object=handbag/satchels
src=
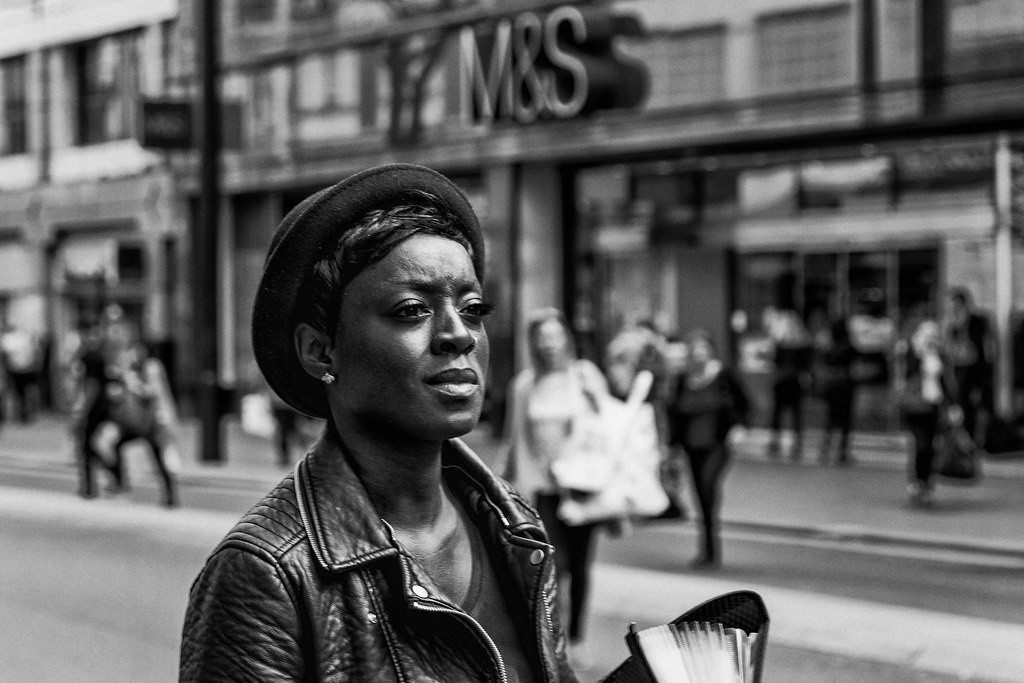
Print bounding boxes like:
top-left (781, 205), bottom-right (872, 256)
top-left (938, 429), bottom-right (980, 480)
top-left (552, 400), bottom-right (668, 528)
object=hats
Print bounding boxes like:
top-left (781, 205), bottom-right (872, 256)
top-left (251, 167), bottom-right (484, 419)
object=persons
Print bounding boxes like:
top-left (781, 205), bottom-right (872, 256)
top-left (178, 163), bottom-right (581, 683)
top-left (270, 392), bottom-right (320, 468)
top-left (632, 285), bottom-right (1022, 575)
top-left (68, 319), bottom-right (181, 509)
top-left (499, 307), bottom-right (613, 673)
